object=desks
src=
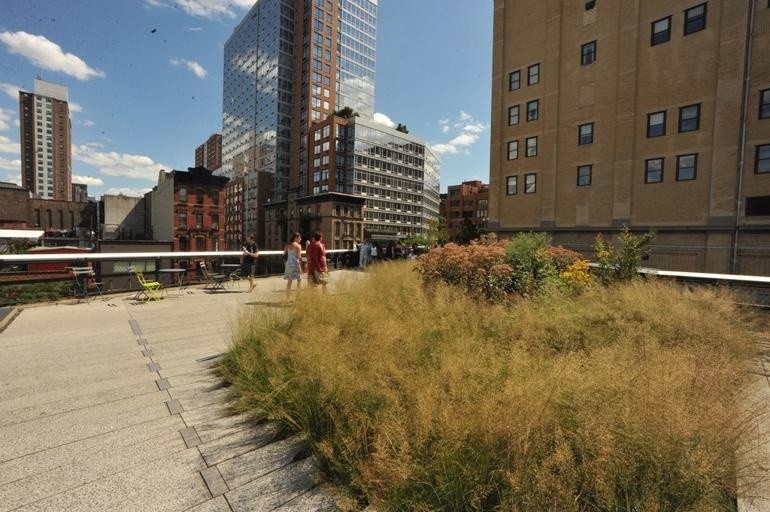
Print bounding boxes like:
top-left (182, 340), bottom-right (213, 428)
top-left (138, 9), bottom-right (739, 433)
top-left (158, 268), bottom-right (188, 299)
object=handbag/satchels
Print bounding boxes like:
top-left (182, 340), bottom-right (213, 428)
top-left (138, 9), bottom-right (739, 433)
top-left (240, 255), bottom-right (246, 264)
top-left (314, 271), bottom-right (329, 285)
top-left (283, 247), bottom-right (288, 261)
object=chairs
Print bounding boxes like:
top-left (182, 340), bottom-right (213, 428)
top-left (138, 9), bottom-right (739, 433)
top-left (124, 266), bottom-right (162, 304)
top-left (197, 260), bottom-right (242, 293)
top-left (64, 265), bottom-right (118, 309)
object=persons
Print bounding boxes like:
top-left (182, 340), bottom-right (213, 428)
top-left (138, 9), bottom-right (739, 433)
top-left (306, 232), bottom-right (329, 289)
top-left (357, 237), bottom-right (422, 269)
top-left (283, 232), bottom-right (303, 304)
top-left (242, 234), bottom-right (259, 294)
